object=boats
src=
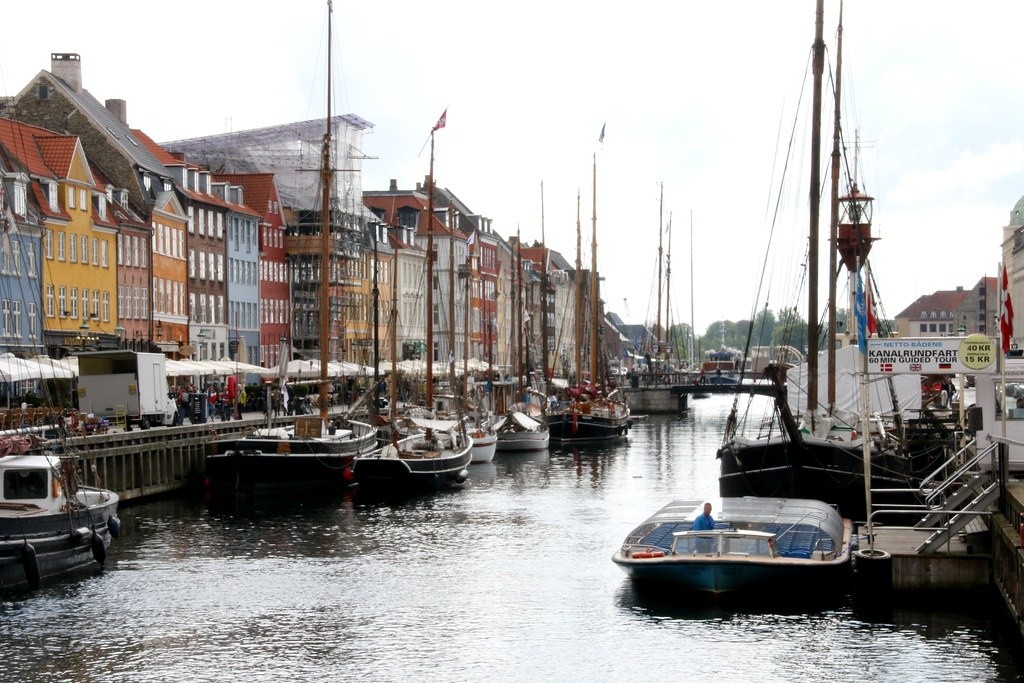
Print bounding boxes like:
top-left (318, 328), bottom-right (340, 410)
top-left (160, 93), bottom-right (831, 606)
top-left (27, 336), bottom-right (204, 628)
top-left (612, 495), bottom-right (855, 616)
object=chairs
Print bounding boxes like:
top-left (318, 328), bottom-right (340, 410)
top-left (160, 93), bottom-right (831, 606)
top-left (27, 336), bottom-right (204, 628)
top-left (0, 406), bottom-right (67, 431)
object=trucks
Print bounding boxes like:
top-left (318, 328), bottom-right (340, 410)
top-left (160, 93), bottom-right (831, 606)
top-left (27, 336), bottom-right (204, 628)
top-left (72, 349), bottom-right (180, 431)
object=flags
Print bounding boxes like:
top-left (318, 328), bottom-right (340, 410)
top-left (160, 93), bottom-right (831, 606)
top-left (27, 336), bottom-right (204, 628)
top-left (999, 265), bottom-right (1015, 354)
top-left (599, 124), bottom-right (605, 143)
top-left (855, 264), bottom-right (879, 353)
top-left (433, 110), bottom-right (445, 131)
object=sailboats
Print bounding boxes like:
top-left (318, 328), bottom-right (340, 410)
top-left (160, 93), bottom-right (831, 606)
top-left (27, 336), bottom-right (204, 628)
top-left (204, 3), bottom-right (379, 519)
top-left (0, 148), bottom-right (122, 593)
top-left (349, 148), bottom-right (711, 466)
top-left (715, 2), bottom-right (924, 526)
top-left (350, 125), bottom-right (474, 505)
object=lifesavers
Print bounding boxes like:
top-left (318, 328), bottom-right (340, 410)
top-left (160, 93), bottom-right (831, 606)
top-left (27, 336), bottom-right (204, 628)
top-left (53, 478), bottom-right (61, 498)
top-left (610, 402), bottom-right (615, 413)
top-left (632, 551), bottom-right (665, 558)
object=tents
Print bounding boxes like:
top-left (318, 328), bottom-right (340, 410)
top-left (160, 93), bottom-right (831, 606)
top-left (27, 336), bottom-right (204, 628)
top-left (0, 357), bottom-right (501, 408)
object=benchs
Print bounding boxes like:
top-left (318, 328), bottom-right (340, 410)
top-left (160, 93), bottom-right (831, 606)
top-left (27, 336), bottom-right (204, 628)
top-left (399, 450), bottom-right (442, 459)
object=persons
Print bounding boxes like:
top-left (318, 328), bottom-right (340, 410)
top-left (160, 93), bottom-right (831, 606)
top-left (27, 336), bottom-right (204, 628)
top-left (692, 503), bottom-right (715, 553)
top-left (942, 377), bottom-right (955, 408)
top-left (714, 368), bottom-right (723, 386)
top-left (171, 378), bottom-right (418, 425)
top-left (699, 368), bottom-right (707, 384)
